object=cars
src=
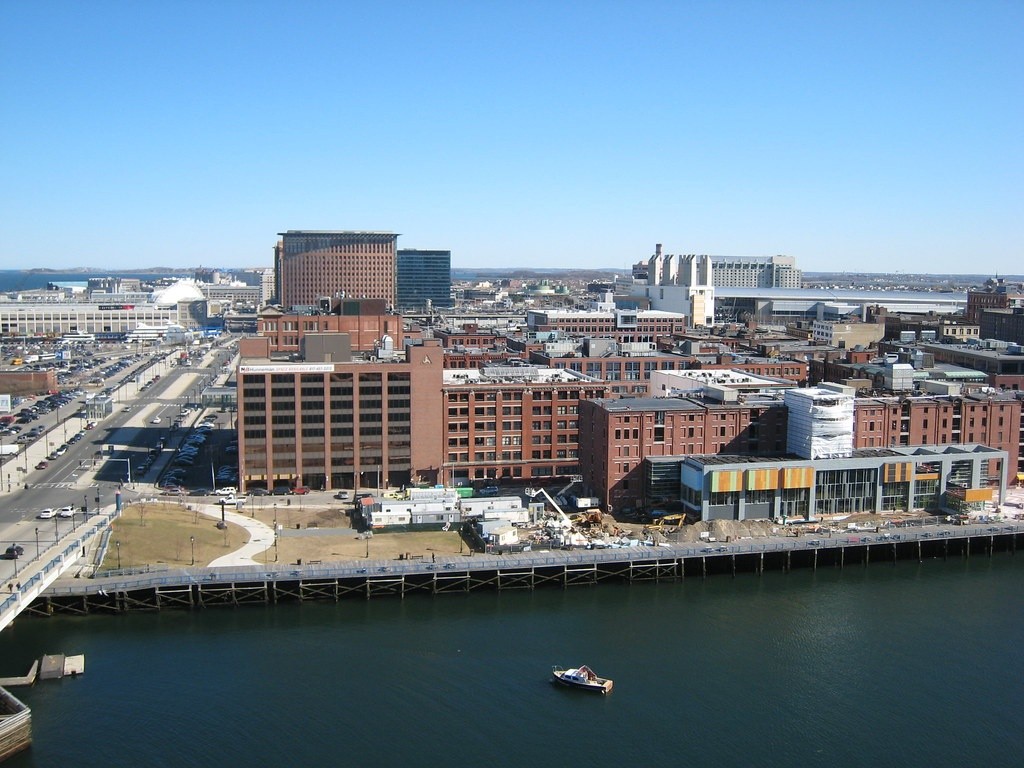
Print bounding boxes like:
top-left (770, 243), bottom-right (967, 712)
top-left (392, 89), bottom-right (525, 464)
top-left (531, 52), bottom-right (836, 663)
top-left (60, 504), bottom-right (76, 520)
top-left (0, 349), bottom-right (239, 496)
top-left (338, 490), bottom-right (349, 499)
top-left (4, 545), bottom-right (24, 560)
top-left (39, 505), bottom-right (55, 518)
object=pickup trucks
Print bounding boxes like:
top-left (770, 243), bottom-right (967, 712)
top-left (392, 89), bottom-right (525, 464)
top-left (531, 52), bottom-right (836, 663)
top-left (219, 494), bottom-right (247, 505)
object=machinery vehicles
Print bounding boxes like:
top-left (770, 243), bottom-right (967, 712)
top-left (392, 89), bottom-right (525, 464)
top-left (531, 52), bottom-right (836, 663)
top-left (523, 486), bottom-right (573, 530)
top-left (643, 513), bottom-right (687, 533)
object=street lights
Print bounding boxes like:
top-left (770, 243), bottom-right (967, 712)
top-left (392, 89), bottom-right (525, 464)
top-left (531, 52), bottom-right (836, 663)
top-left (54, 514), bottom-right (59, 543)
top-left (95, 488), bottom-right (100, 515)
top-left (84, 495), bottom-right (88, 523)
top-left (71, 502), bottom-right (76, 532)
top-left (12, 543), bottom-right (18, 577)
top-left (273, 503), bottom-right (278, 563)
top-left (116, 541), bottom-right (120, 570)
top-left (190, 536), bottom-right (195, 565)
top-left (36, 527), bottom-right (39, 561)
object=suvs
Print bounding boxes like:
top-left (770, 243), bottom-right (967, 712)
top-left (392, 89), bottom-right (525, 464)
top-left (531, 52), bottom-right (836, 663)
top-left (214, 486), bottom-right (236, 496)
top-left (247, 485), bottom-right (310, 498)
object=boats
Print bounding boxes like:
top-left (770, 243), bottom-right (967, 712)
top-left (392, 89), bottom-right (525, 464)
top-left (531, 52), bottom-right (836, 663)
top-left (552, 667), bottom-right (615, 696)
